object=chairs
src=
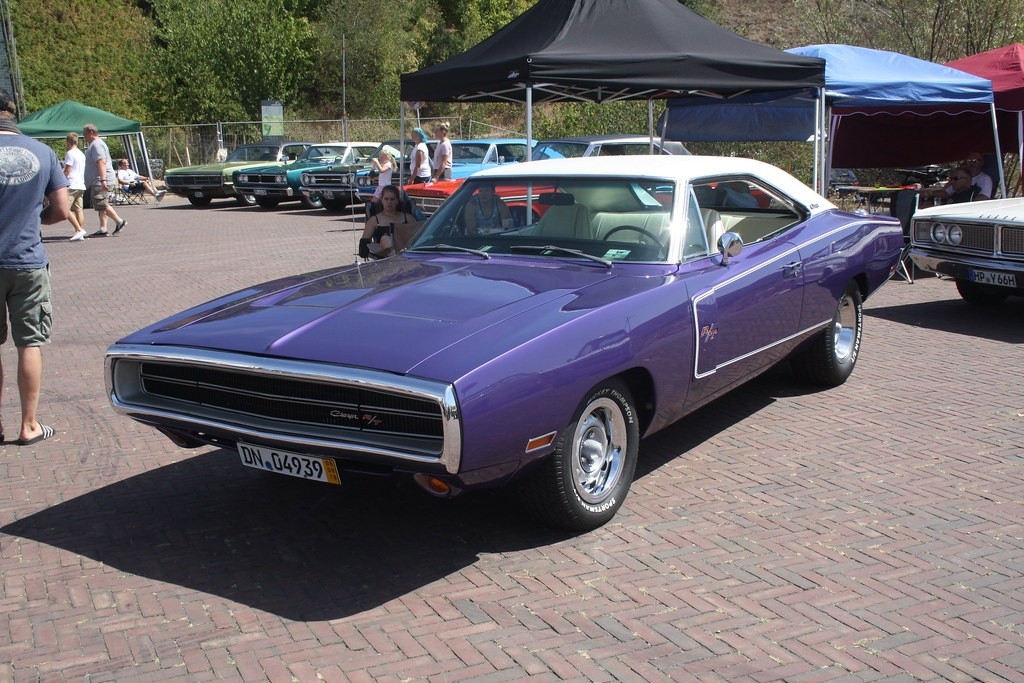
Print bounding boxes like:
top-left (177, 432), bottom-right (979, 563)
top-left (359, 191), bottom-right (426, 263)
top-left (115, 171), bottom-right (148, 205)
top-left (892, 153), bottom-right (1008, 286)
top-left (661, 209), bottom-right (727, 264)
top-left (530, 205), bottom-right (592, 240)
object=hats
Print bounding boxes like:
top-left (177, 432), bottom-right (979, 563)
top-left (0, 88), bottom-right (17, 114)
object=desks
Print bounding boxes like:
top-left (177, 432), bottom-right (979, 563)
top-left (856, 186), bottom-right (909, 213)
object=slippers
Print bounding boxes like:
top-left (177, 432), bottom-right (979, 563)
top-left (17, 421), bottom-right (56, 445)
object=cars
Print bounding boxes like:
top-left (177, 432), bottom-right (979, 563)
top-left (908, 194), bottom-right (1024, 318)
top-left (100, 154), bottom-right (903, 535)
top-left (353, 138), bottom-right (566, 204)
top-left (829, 168), bottom-right (861, 197)
top-left (163, 142), bottom-right (337, 207)
top-left (231, 142), bottom-right (408, 209)
top-left (397, 135), bottom-right (771, 231)
top-left (298, 139), bottom-right (415, 214)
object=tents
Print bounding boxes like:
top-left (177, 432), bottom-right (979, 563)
top-left (944, 41), bottom-right (1024, 177)
top-left (12, 98), bottom-right (160, 204)
top-left (398, 0), bottom-right (827, 225)
top-left (656, 43), bottom-right (1007, 198)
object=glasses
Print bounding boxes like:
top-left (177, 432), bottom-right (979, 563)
top-left (966, 159), bottom-right (976, 165)
top-left (949, 177), bottom-right (967, 182)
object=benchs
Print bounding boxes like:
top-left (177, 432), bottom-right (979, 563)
top-left (593, 210), bottom-right (789, 261)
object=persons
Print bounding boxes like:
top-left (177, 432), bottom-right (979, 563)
top-left (465, 183), bottom-right (512, 235)
top-left (370, 150), bottom-right (398, 202)
top-left (932, 147), bottom-right (993, 206)
top-left (62, 124), bottom-right (128, 241)
top-left (117, 159), bottom-right (166, 202)
top-left (0, 87), bottom-right (70, 445)
top-left (359, 185), bottom-right (418, 259)
top-left (432, 121), bottom-right (452, 180)
top-left (407, 127), bottom-right (431, 184)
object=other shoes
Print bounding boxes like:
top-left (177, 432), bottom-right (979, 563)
top-left (112, 220), bottom-right (126, 236)
top-left (70, 234), bottom-right (84, 241)
top-left (90, 230), bottom-right (109, 238)
top-left (157, 190), bottom-right (167, 202)
